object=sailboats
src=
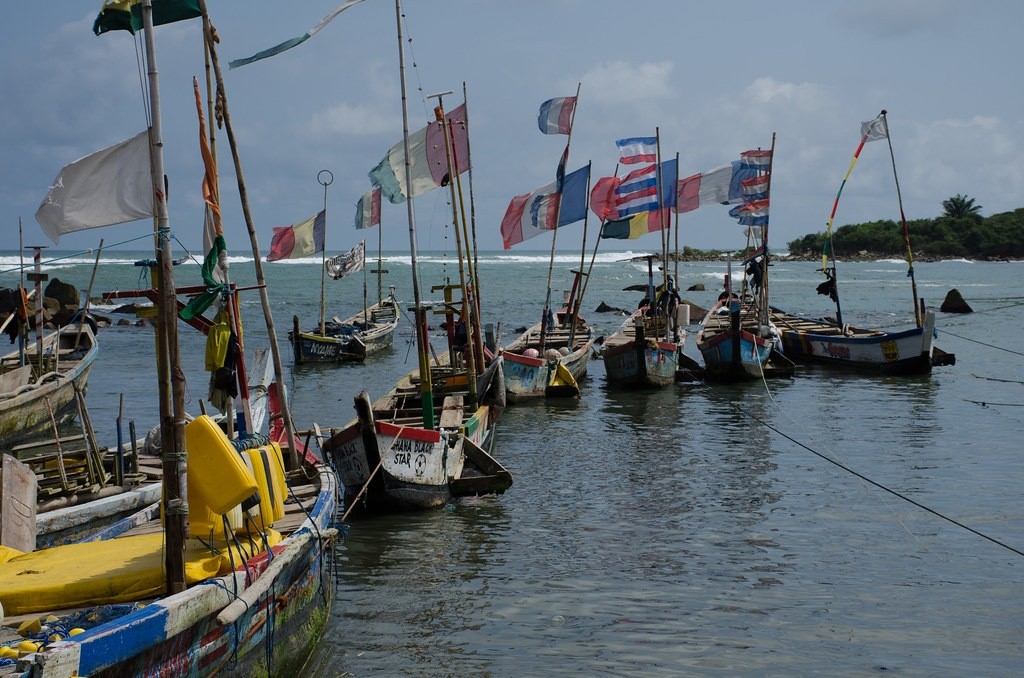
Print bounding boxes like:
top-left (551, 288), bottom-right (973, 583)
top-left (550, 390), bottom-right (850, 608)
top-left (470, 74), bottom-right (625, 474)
top-left (502, 83), bottom-right (619, 405)
top-left (322, 0), bottom-right (513, 514)
top-left (598, 127), bottom-right (686, 392)
top-left (695, 132), bottom-right (784, 382)
top-left (0, 0), bottom-right (338, 678)
top-left (767, 109), bottom-right (937, 378)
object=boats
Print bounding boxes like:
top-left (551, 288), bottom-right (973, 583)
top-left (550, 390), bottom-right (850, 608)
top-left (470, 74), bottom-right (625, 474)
top-left (267, 170), bottom-right (399, 366)
top-left (87, 302), bottom-right (154, 328)
top-left (0, 216), bottom-right (99, 449)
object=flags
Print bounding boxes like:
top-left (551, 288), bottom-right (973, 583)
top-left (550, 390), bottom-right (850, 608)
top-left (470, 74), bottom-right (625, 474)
top-left (368, 103), bottom-right (470, 204)
top-left (743, 226), bottom-right (762, 239)
top-left (34, 127), bottom-right (154, 245)
top-left (737, 199), bottom-right (769, 225)
top-left (861, 115), bottom-right (888, 143)
top-left (176, 88), bottom-right (240, 416)
top-left (538, 95), bottom-right (577, 135)
top-left (500, 163), bottom-right (590, 251)
top-left (556, 145), bottom-right (568, 193)
top-left (92, 0), bottom-right (204, 37)
top-left (740, 174), bottom-right (769, 202)
top-left (728, 203), bottom-right (747, 218)
top-left (326, 238), bottom-right (365, 280)
top-left (615, 137), bottom-right (657, 164)
top-left (741, 244), bottom-right (764, 267)
top-left (740, 149), bottom-right (771, 171)
top-left (671, 159), bottom-right (757, 214)
top-left (591, 157), bottom-right (676, 223)
top-left (600, 198), bottom-right (670, 240)
top-left (354, 186), bottom-right (379, 230)
top-left (266, 211), bottom-right (324, 262)
